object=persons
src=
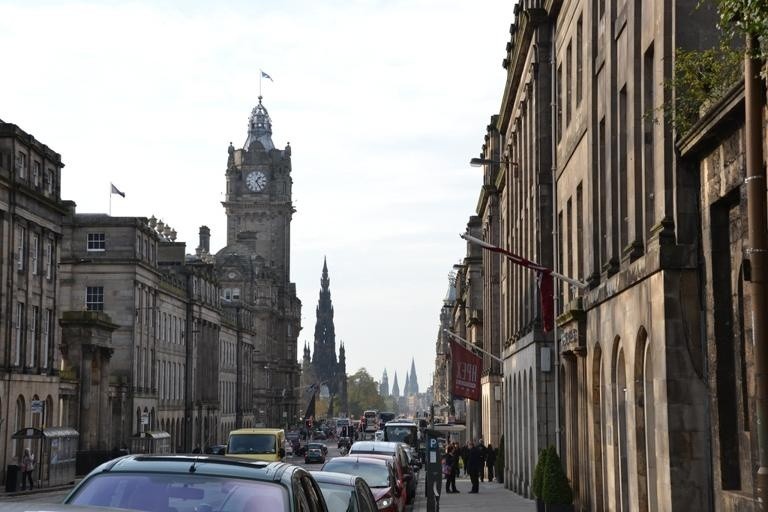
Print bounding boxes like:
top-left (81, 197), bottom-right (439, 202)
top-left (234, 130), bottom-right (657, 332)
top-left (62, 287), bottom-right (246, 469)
top-left (443, 438), bottom-right (496, 494)
top-left (21, 446), bottom-right (35, 491)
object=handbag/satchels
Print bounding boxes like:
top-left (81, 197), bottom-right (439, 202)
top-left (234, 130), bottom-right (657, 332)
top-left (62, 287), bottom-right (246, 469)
top-left (442, 464), bottom-right (452, 475)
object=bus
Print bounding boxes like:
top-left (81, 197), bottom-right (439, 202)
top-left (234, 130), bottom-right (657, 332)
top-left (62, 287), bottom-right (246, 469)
top-left (224, 428), bottom-right (286, 462)
top-left (380, 412), bottom-right (428, 451)
top-left (363, 411), bottom-right (378, 432)
top-left (336, 419), bottom-right (351, 437)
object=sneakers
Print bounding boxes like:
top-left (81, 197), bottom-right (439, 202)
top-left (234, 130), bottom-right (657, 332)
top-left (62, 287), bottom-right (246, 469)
top-left (468, 489), bottom-right (479, 494)
top-left (446, 488), bottom-right (460, 494)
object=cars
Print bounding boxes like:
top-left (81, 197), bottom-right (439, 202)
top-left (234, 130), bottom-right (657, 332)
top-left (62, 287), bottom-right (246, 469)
top-left (310, 442), bottom-right (422, 512)
top-left (287, 433), bottom-right (328, 463)
top-left (63, 452), bottom-right (328, 512)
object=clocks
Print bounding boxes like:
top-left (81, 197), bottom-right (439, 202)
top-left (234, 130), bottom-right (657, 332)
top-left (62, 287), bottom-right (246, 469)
top-left (245, 169), bottom-right (268, 193)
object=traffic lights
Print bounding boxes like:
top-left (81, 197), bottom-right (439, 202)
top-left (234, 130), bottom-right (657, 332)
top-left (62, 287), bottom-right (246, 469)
top-left (305, 420), bottom-right (312, 428)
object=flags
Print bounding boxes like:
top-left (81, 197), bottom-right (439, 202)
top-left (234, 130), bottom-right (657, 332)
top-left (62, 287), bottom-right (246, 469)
top-left (111, 183), bottom-right (126, 199)
top-left (449, 338), bottom-right (482, 402)
top-left (262, 71), bottom-right (273, 83)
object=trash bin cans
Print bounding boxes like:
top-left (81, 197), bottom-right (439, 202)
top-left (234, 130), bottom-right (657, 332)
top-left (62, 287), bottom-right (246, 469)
top-left (6, 465), bottom-right (23, 492)
